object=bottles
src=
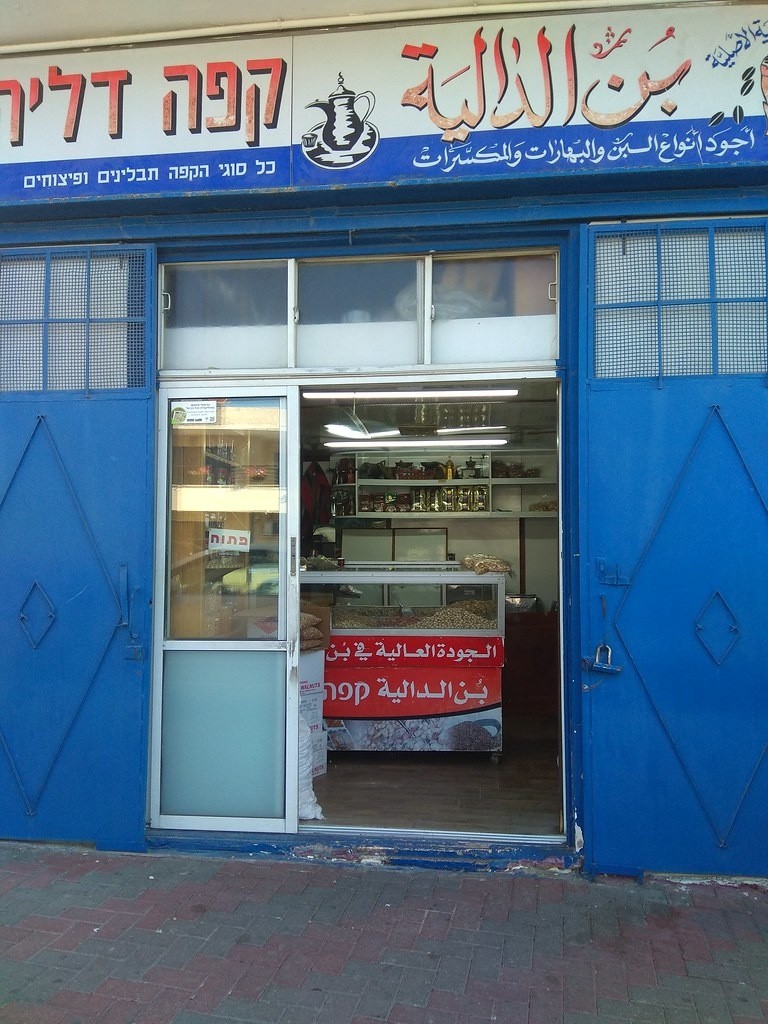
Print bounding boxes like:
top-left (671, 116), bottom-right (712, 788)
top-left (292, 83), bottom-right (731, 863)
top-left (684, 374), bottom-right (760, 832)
top-left (445, 456), bottom-right (453, 480)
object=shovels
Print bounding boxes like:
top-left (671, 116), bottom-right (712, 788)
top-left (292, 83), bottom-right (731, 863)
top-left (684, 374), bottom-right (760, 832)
top-left (392, 591), bottom-right (414, 617)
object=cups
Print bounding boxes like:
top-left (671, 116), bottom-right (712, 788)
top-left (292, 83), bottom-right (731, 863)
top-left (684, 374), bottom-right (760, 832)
top-left (338, 558), bottom-right (345, 567)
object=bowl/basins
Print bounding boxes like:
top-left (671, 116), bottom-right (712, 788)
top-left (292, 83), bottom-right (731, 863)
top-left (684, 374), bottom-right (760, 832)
top-left (465, 461), bottom-right (476, 467)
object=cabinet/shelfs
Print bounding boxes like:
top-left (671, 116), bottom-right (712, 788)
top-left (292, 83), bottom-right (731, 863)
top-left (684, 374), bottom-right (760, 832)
top-left (327, 449), bottom-right (559, 520)
top-left (167, 429), bottom-right (252, 642)
